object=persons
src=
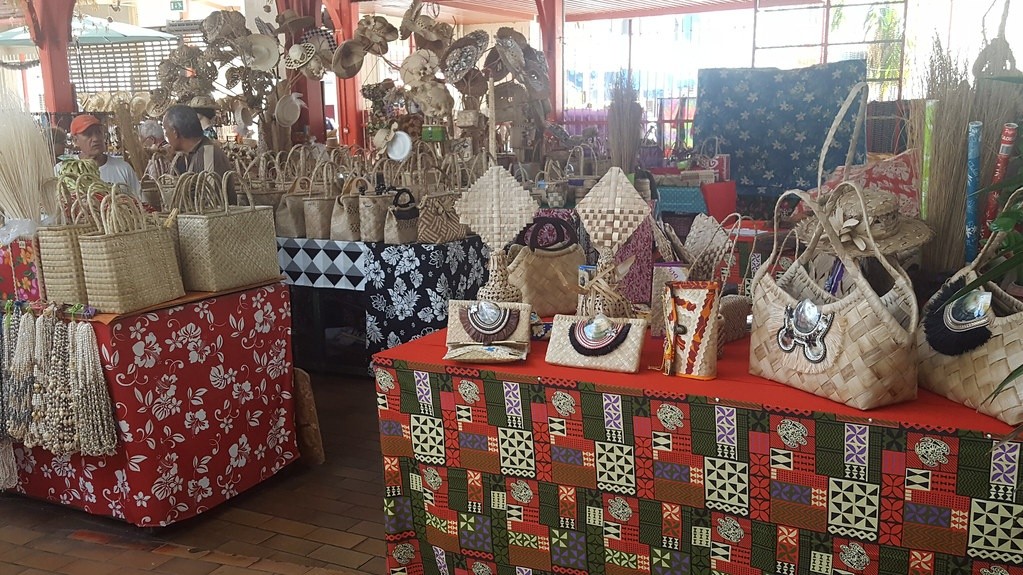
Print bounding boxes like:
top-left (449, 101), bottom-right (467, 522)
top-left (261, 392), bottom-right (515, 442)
top-left (42, 126), bottom-right (67, 165)
top-left (139, 120), bottom-right (171, 149)
top-left (54, 115), bottom-right (144, 204)
top-left (163, 104), bottom-right (238, 206)
top-left (188, 95), bottom-right (218, 142)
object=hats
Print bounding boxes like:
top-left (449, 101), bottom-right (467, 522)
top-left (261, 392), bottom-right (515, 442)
top-left (85, 8), bottom-right (556, 161)
top-left (70, 115), bottom-right (101, 135)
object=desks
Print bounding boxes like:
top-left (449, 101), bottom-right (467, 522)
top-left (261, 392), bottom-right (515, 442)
top-left (0, 181), bottom-right (1023, 575)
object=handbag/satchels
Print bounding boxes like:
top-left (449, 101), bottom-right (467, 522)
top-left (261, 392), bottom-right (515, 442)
top-left (253, 82), bottom-right (1023, 427)
top-left (38, 172), bottom-right (279, 316)
